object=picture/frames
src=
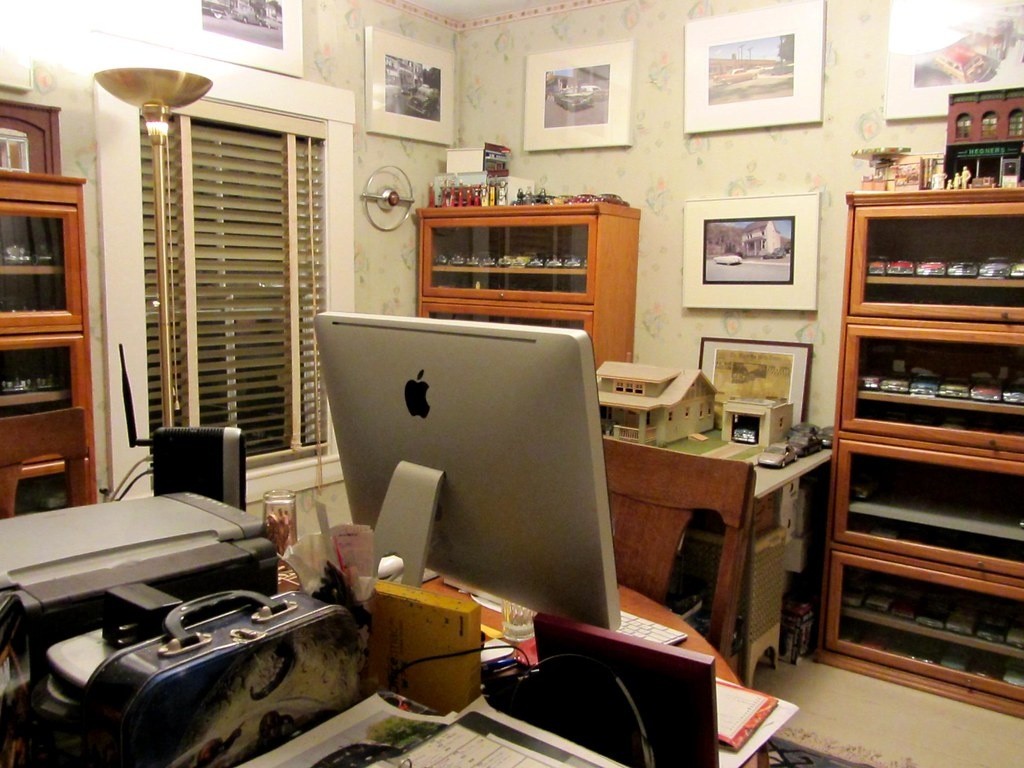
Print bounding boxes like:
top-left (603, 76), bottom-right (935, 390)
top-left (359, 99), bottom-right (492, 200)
top-left (684, 2), bottom-right (828, 134)
top-left (523, 37), bottom-right (635, 151)
top-left (698, 337), bottom-right (813, 428)
top-left (200, 0), bottom-right (302, 78)
top-left (683, 193), bottom-right (818, 310)
top-left (364, 25), bottom-right (452, 145)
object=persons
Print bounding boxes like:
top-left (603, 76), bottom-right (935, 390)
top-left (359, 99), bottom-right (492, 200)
top-left (946, 166), bottom-right (971, 189)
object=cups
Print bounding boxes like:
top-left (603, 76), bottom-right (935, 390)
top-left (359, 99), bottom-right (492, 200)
top-left (261, 489), bottom-right (298, 570)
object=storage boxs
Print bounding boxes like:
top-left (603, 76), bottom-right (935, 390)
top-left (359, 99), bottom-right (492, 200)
top-left (777, 597), bottom-right (817, 664)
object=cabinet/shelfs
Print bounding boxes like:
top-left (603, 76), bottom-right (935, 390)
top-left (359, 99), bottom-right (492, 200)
top-left (414, 201), bottom-right (641, 374)
top-left (816, 187), bottom-right (1024, 716)
top-left (0, 168), bottom-right (98, 509)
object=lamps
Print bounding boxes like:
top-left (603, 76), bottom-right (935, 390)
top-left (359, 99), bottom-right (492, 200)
top-left (97, 67), bottom-right (215, 425)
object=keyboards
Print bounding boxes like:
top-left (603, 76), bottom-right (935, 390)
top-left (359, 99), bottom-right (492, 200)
top-left (442, 577), bottom-right (688, 648)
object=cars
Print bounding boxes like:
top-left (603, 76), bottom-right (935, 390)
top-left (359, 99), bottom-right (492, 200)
top-left (859, 372), bottom-right (1024, 404)
top-left (841, 594), bottom-right (1024, 651)
top-left (202, 0), bottom-right (282, 31)
top-left (510, 193), bottom-right (630, 206)
top-left (757, 246), bottom-right (786, 260)
top-left (869, 255), bottom-right (1024, 278)
top-left (405, 83), bottom-right (440, 119)
top-left (714, 66), bottom-right (767, 84)
top-left (713, 253), bottom-right (742, 265)
top-left (554, 85), bottom-right (601, 113)
top-left (434, 254), bottom-right (587, 269)
top-left (757, 424), bottom-right (834, 469)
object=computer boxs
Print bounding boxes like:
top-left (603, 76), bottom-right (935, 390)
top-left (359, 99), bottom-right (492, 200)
top-left (151, 426), bottom-right (246, 512)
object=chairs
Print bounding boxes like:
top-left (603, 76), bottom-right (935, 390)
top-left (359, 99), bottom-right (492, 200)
top-left (2, 408), bottom-right (90, 518)
top-left (110, 342), bottom-right (154, 500)
top-left (603, 435), bottom-right (756, 673)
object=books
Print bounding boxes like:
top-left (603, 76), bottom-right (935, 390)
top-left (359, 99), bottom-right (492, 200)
top-left (716, 681), bottom-right (778, 752)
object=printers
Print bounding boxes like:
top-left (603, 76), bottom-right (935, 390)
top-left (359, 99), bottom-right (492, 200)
top-left (0, 492), bottom-right (280, 738)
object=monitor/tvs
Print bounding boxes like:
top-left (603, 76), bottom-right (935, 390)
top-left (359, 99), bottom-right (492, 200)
top-left (314, 310), bottom-right (622, 635)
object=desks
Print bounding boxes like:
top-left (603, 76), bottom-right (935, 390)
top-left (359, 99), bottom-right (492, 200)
top-left (276, 540), bottom-right (770, 768)
top-left (655, 427), bottom-right (834, 675)
